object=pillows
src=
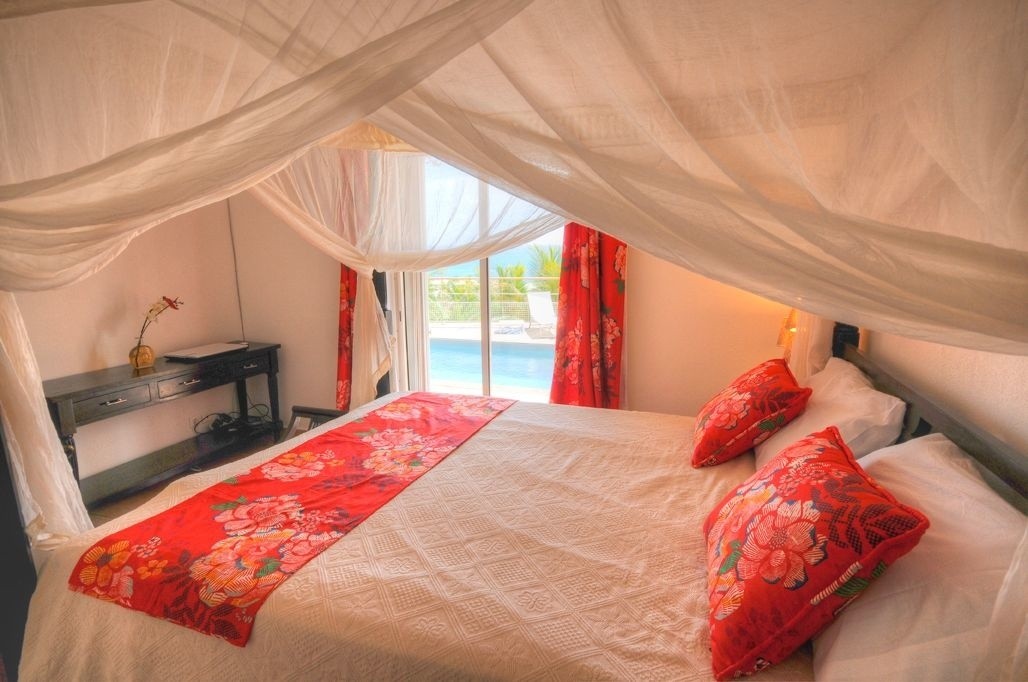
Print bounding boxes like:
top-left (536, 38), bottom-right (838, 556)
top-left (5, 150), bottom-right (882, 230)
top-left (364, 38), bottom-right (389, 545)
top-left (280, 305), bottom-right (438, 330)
top-left (701, 426), bottom-right (929, 681)
top-left (752, 357), bottom-right (908, 478)
top-left (692, 357), bottom-right (813, 469)
top-left (809, 433), bottom-right (1027, 682)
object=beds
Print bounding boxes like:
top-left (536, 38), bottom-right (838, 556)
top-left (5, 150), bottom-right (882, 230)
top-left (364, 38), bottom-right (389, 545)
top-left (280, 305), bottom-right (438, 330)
top-left (0, 319), bottom-right (1027, 682)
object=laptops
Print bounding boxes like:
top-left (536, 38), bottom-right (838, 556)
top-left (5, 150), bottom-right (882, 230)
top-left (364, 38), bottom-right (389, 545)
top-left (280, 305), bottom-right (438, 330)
top-left (164, 343), bottom-right (248, 361)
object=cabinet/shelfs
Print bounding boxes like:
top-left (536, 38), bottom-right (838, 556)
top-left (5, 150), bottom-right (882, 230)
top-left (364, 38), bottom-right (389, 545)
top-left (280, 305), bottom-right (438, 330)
top-left (39, 340), bottom-right (282, 516)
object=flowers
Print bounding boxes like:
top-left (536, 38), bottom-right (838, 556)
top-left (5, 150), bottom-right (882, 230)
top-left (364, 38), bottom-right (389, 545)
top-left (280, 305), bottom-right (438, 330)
top-left (133, 293), bottom-right (184, 366)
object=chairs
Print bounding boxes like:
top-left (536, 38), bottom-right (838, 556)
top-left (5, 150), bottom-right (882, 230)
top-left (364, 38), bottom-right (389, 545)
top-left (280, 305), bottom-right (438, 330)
top-left (527, 289), bottom-right (557, 339)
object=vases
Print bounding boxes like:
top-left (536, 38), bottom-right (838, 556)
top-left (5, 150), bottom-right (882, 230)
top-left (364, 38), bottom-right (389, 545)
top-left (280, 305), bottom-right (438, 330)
top-left (129, 336), bottom-right (156, 368)
